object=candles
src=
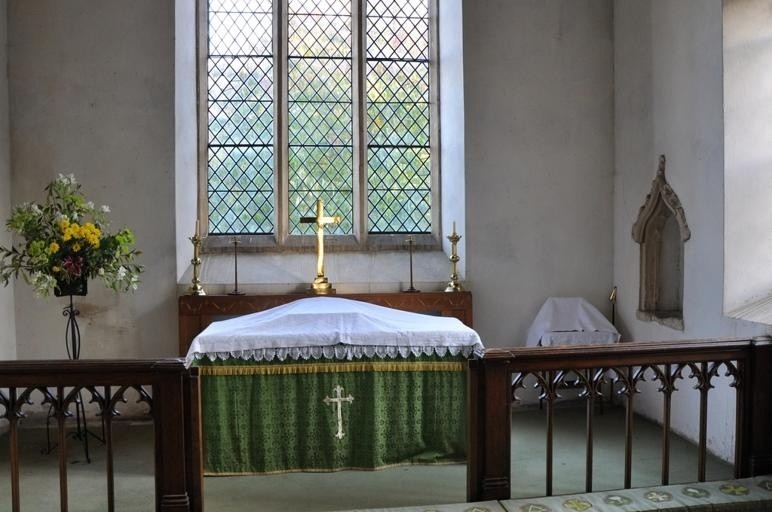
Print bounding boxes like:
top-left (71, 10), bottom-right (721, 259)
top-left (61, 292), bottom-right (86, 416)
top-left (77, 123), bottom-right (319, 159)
top-left (195, 219), bottom-right (200, 237)
top-left (451, 220), bottom-right (457, 235)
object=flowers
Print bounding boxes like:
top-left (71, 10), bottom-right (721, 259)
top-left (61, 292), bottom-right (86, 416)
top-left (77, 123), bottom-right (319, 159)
top-left (0, 170), bottom-right (147, 301)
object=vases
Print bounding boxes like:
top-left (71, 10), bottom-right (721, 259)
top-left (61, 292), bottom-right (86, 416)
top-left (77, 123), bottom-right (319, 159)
top-left (44, 256), bottom-right (95, 298)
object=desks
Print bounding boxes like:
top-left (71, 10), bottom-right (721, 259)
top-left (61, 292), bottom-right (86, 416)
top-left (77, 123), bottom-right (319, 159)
top-left (533, 328), bottom-right (619, 416)
